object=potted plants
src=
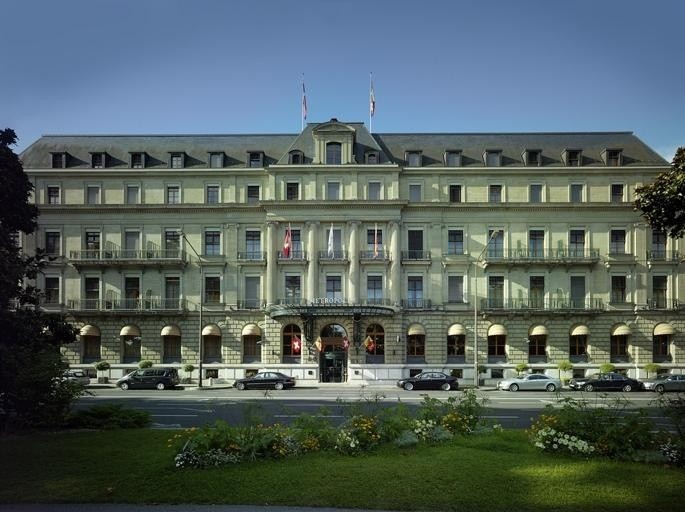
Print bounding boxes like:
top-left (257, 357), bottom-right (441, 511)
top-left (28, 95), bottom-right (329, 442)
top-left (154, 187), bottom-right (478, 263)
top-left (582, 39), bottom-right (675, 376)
top-left (559, 360), bottom-right (573, 386)
top-left (477, 365), bottom-right (487, 387)
top-left (94, 361), bottom-right (110, 384)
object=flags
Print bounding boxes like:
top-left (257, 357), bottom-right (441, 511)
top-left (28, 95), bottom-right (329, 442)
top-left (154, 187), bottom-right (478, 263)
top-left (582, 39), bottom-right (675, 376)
top-left (290, 336), bottom-right (302, 352)
top-left (314, 336), bottom-right (326, 352)
top-left (342, 336), bottom-right (350, 350)
top-left (368, 74), bottom-right (376, 120)
top-left (373, 223), bottom-right (379, 257)
top-left (362, 335), bottom-right (376, 352)
top-left (283, 223), bottom-right (292, 257)
top-left (326, 223), bottom-right (334, 256)
top-left (301, 82), bottom-right (308, 121)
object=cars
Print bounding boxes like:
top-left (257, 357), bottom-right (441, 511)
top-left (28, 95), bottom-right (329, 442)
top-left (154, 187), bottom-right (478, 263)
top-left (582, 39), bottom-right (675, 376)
top-left (232, 370), bottom-right (294, 390)
top-left (57, 368), bottom-right (91, 386)
top-left (641, 374), bottom-right (685, 394)
top-left (569, 370), bottom-right (640, 393)
top-left (498, 373), bottom-right (561, 392)
top-left (396, 372), bottom-right (459, 391)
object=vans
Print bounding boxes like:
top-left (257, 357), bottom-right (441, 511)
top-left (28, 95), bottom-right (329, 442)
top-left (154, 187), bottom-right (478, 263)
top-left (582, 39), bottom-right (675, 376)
top-left (115, 368), bottom-right (180, 391)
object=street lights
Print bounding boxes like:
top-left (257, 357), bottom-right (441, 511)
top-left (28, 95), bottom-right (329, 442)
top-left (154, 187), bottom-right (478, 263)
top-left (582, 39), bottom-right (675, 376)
top-left (175, 227), bottom-right (206, 388)
top-left (470, 226), bottom-right (500, 390)
